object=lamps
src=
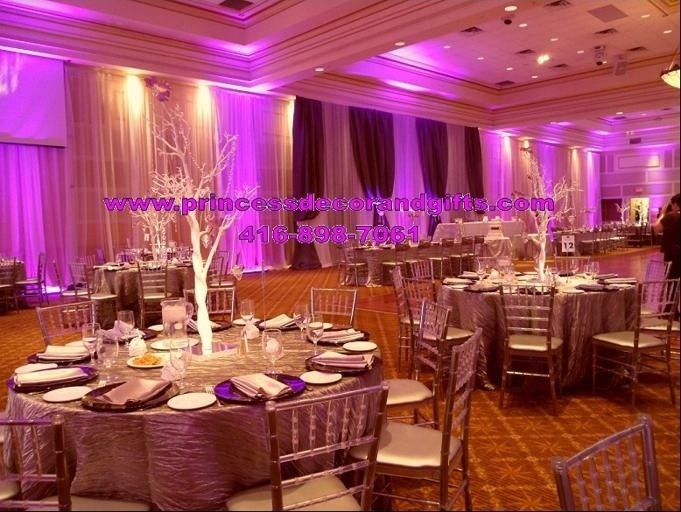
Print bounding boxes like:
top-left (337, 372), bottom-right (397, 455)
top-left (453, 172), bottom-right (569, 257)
top-left (659, 41), bottom-right (679, 88)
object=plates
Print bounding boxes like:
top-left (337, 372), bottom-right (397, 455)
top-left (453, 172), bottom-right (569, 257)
top-left (215, 373), bottom-right (305, 404)
top-left (27, 346), bottom-right (92, 365)
top-left (186, 319), bottom-right (234, 333)
top-left (301, 370), bottom-right (343, 385)
top-left (14, 362), bottom-right (57, 373)
top-left (305, 327), bottom-right (370, 344)
top-left (166, 391), bottom-right (217, 409)
top-left (233, 318), bottom-right (260, 325)
top-left (126, 353), bottom-right (183, 368)
top-left (459, 273), bottom-right (480, 280)
top-left (42, 384), bottom-right (93, 404)
top-left (147, 322), bottom-right (183, 332)
top-left (342, 340), bottom-right (379, 352)
top-left (150, 337), bottom-right (199, 350)
top-left (304, 321), bottom-right (334, 330)
top-left (6, 365), bottom-right (97, 395)
top-left (305, 352), bottom-right (383, 374)
top-left (82, 378), bottom-right (179, 413)
top-left (254, 317), bottom-right (299, 331)
top-left (466, 282), bottom-right (501, 292)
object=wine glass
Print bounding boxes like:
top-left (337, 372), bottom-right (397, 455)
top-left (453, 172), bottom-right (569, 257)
top-left (117, 310), bottom-right (135, 368)
top-left (307, 319), bottom-right (324, 355)
top-left (293, 304), bottom-right (311, 343)
top-left (261, 329), bottom-right (286, 371)
top-left (101, 248), bottom-right (195, 268)
top-left (169, 336), bottom-right (192, 395)
top-left (580, 220), bottom-right (634, 232)
top-left (240, 299), bottom-right (255, 327)
top-left (476, 251), bottom-right (638, 294)
top-left (82, 323), bottom-right (101, 364)
top-left (96, 334), bottom-right (118, 375)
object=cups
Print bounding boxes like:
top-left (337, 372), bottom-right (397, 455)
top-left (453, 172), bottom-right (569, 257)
top-left (161, 297), bottom-right (194, 338)
top-left (483, 214), bottom-right (502, 222)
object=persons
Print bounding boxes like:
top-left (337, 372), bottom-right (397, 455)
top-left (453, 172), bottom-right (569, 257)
top-left (654, 193), bottom-right (680, 321)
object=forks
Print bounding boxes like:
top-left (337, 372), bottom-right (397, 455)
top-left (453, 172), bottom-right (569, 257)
top-left (202, 382), bottom-right (225, 408)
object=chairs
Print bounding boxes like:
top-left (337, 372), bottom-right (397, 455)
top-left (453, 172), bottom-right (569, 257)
top-left (1, 224), bottom-right (681, 511)
